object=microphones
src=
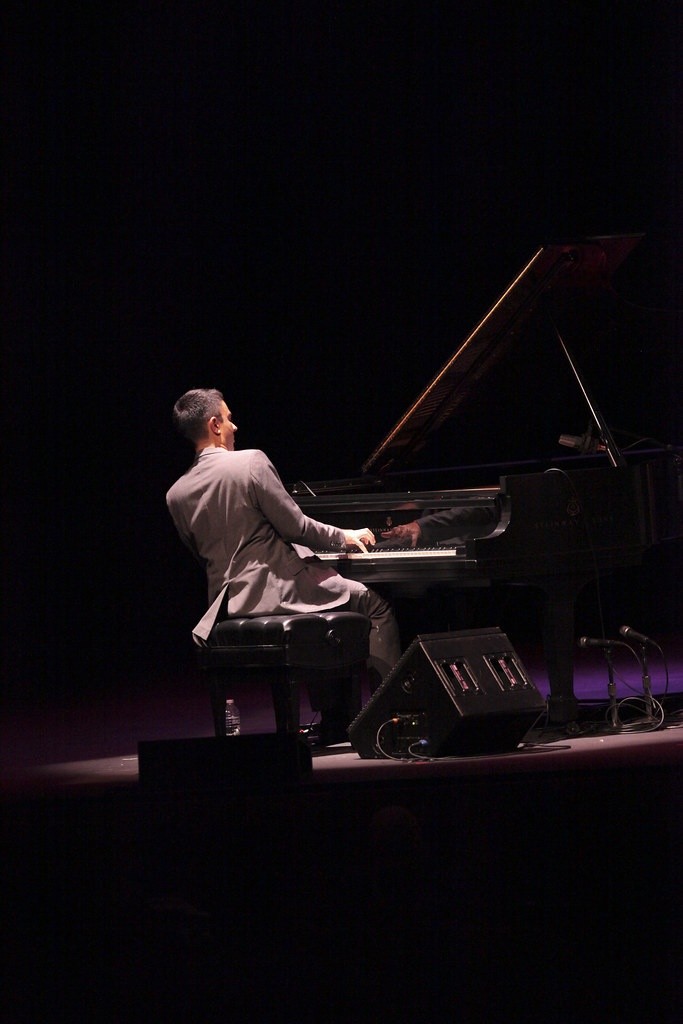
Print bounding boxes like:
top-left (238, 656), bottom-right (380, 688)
top-left (559, 433), bottom-right (609, 456)
top-left (619, 626), bottom-right (658, 647)
top-left (580, 636), bottom-right (626, 649)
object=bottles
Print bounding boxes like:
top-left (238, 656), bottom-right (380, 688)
top-left (226, 699), bottom-right (240, 736)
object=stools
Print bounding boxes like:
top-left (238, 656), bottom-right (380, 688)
top-left (197, 609), bottom-right (372, 753)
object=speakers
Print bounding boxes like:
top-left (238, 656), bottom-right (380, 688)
top-left (346, 627), bottom-right (549, 760)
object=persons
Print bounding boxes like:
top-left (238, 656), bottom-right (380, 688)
top-left (382, 505), bottom-right (491, 548)
top-left (165, 389), bottom-right (400, 711)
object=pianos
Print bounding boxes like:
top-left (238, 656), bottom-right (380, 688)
top-left (282, 232), bottom-right (683, 737)
